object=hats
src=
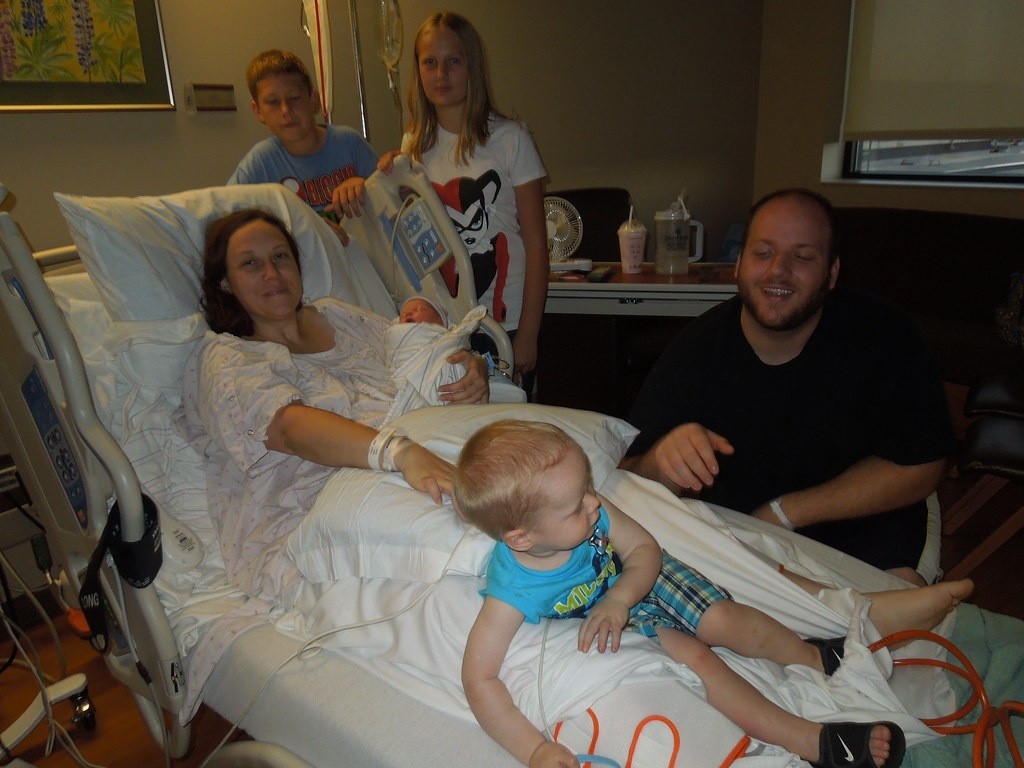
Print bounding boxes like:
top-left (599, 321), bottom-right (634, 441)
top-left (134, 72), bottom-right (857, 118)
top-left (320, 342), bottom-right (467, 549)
top-left (400, 295), bottom-right (449, 330)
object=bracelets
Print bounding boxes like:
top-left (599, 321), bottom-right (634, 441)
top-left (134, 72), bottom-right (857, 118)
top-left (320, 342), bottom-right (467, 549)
top-left (368, 427), bottom-right (411, 471)
top-left (770, 495), bottom-right (795, 531)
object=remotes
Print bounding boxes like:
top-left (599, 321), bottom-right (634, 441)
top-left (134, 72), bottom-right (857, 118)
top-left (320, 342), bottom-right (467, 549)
top-left (550, 258), bottom-right (592, 272)
top-left (586, 266), bottom-right (611, 283)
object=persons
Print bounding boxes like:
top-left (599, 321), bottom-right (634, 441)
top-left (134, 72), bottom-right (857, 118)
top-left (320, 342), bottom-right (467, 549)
top-left (385, 293), bottom-right (490, 406)
top-left (379, 12), bottom-right (549, 389)
top-left (227, 50), bottom-right (378, 247)
top-left (624, 190), bottom-right (948, 590)
top-left (184, 212), bottom-right (973, 653)
top-left (454, 420), bottom-right (908, 768)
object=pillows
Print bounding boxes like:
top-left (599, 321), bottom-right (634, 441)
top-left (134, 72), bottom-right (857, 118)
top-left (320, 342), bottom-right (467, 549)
top-left (54, 182), bottom-right (366, 409)
top-left (259, 400), bottom-right (641, 586)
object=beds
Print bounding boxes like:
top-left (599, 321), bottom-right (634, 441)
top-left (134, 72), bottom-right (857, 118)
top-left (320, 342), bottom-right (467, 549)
top-left (0, 155), bottom-right (1024, 768)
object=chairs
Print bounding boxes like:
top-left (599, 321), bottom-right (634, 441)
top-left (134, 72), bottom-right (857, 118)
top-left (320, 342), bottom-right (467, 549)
top-left (542, 187), bottom-right (639, 270)
top-left (953, 346), bottom-right (1024, 482)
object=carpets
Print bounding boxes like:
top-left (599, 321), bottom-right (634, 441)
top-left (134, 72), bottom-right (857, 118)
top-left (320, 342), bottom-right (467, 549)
top-left (920, 379), bottom-right (1024, 591)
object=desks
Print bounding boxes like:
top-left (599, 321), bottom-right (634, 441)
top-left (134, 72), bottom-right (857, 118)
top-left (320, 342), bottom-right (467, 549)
top-left (528, 262), bottom-right (744, 397)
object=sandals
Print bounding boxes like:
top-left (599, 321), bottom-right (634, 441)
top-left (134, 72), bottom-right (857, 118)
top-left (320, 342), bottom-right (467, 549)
top-left (800, 721), bottom-right (906, 768)
top-left (803, 636), bottom-right (846, 676)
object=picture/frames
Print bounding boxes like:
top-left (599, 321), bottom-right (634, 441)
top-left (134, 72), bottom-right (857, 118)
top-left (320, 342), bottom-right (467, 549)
top-left (0, 0), bottom-right (177, 114)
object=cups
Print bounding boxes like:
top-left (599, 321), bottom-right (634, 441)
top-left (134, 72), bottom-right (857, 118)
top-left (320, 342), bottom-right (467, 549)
top-left (653, 210), bottom-right (704, 276)
top-left (618, 220), bottom-right (647, 274)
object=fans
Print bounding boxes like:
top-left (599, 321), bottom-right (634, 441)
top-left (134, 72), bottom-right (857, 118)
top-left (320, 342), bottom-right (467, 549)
top-left (542, 195), bottom-right (584, 263)
